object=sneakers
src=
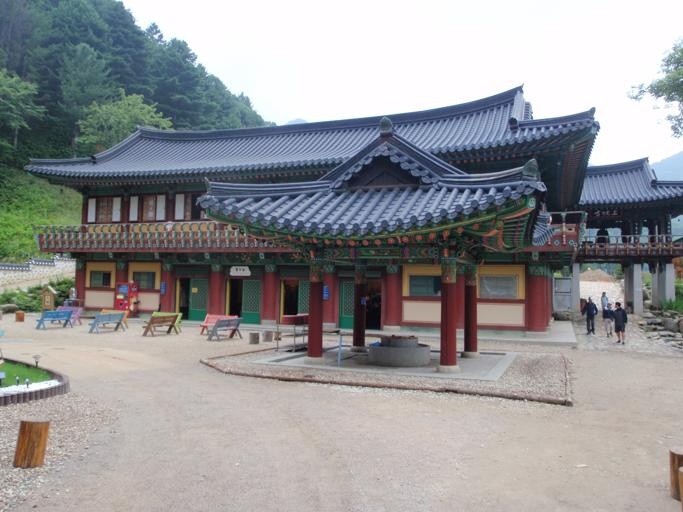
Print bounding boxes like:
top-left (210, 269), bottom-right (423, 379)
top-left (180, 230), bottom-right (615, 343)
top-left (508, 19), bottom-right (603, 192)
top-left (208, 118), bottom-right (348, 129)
top-left (586, 329), bottom-right (625, 345)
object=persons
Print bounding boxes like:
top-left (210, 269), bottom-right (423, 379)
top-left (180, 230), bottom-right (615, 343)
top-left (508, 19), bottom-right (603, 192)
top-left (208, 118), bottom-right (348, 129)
top-left (600, 292), bottom-right (608, 309)
top-left (602, 303), bottom-right (612, 337)
top-left (609, 301), bottom-right (627, 345)
top-left (582, 297), bottom-right (597, 336)
top-left (595, 225), bottom-right (609, 247)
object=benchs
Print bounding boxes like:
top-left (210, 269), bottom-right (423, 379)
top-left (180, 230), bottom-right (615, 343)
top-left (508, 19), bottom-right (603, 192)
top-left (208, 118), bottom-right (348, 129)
top-left (29, 301), bottom-right (242, 346)
